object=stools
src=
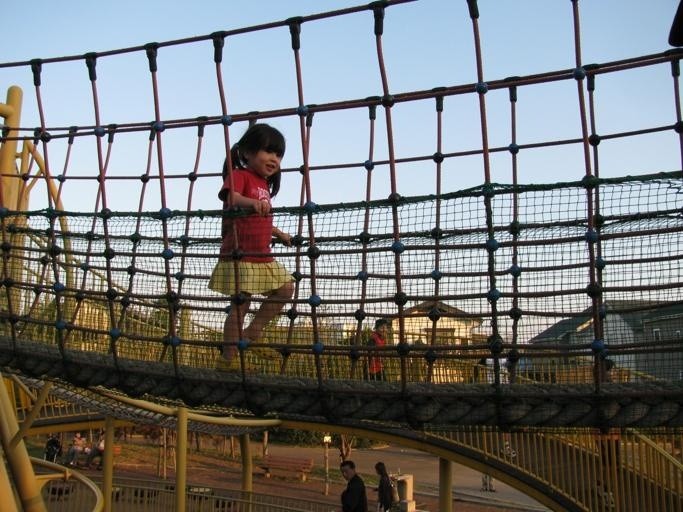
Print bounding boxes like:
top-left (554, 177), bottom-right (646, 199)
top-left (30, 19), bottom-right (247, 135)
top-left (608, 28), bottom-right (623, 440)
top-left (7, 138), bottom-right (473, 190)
top-left (48, 480), bottom-right (237, 508)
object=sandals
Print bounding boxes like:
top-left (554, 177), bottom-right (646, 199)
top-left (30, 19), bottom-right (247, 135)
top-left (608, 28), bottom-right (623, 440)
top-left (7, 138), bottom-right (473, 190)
top-left (216, 338), bottom-right (275, 371)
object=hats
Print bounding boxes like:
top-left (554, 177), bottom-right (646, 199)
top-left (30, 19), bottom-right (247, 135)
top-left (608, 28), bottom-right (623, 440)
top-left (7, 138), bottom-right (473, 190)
top-left (373, 319), bottom-right (392, 329)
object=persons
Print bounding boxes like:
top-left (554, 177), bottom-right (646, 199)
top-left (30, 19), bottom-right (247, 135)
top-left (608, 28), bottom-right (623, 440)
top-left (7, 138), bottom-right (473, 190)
top-left (479, 471), bottom-right (496, 492)
top-left (60, 430), bottom-right (84, 468)
top-left (469, 330), bottom-right (521, 462)
top-left (338, 460), bottom-right (367, 511)
top-left (374, 460), bottom-right (394, 511)
top-left (360, 317), bottom-right (389, 381)
top-left (208, 122), bottom-right (297, 370)
top-left (584, 359), bottom-right (621, 511)
top-left (85, 427), bottom-right (107, 471)
top-left (40, 432), bottom-right (59, 463)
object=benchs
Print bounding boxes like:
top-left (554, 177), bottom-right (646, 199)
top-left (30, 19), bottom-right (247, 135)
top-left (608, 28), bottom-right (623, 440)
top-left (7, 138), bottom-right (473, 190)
top-left (63, 446), bottom-right (120, 468)
top-left (253, 455), bottom-right (315, 481)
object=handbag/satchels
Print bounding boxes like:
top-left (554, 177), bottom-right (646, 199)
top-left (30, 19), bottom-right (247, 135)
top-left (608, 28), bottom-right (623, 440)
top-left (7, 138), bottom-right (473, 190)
top-left (390, 481), bottom-right (400, 504)
